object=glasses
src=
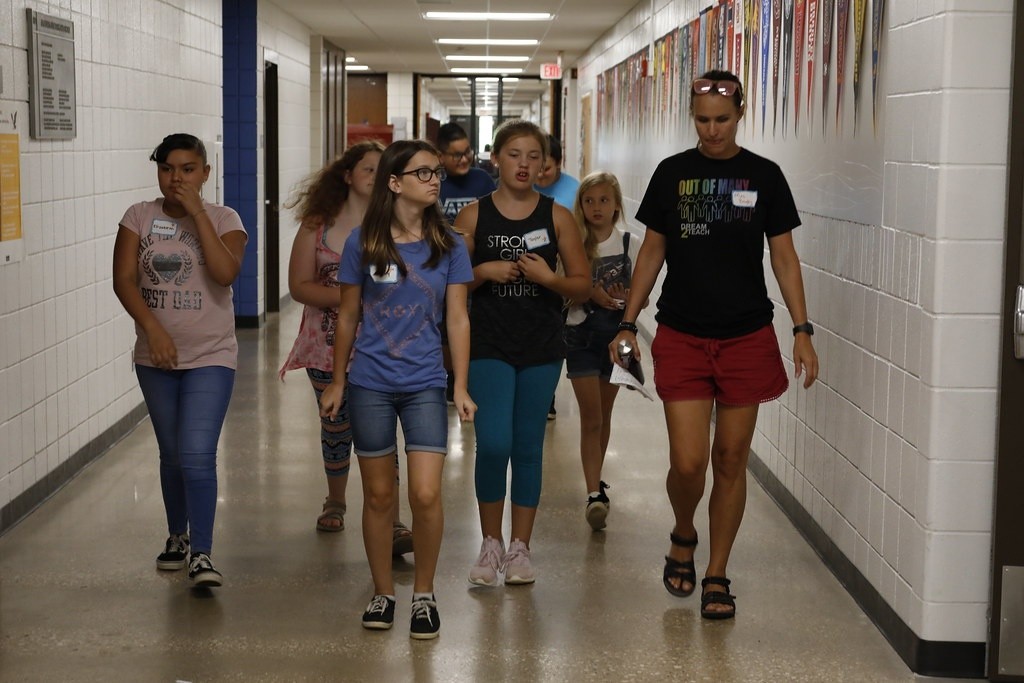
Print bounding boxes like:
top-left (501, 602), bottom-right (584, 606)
top-left (442, 146), bottom-right (472, 162)
top-left (400, 167), bottom-right (447, 182)
top-left (691, 78), bottom-right (745, 104)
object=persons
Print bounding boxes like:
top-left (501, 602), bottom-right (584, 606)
top-left (609, 70), bottom-right (819, 613)
top-left (433, 122), bottom-right (498, 406)
top-left (112, 134), bottom-right (247, 588)
top-left (319, 139), bottom-right (476, 639)
top-left (533, 131), bottom-right (581, 421)
top-left (557, 171), bottom-right (650, 530)
top-left (452, 121), bottom-right (594, 586)
top-left (278, 141), bottom-right (414, 557)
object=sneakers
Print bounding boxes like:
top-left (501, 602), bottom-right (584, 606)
top-left (468, 535), bottom-right (505, 586)
top-left (410, 593), bottom-right (440, 639)
top-left (156, 532), bottom-right (190, 570)
top-left (499, 538), bottom-right (535, 584)
top-left (188, 552), bottom-right (223, 587)
top-left (362, 595), bottom-right (396, 629)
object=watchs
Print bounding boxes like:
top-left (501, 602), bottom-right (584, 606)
top-left (793, 322), bottom-right (814, 335)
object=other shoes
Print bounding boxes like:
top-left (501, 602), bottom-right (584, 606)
top-left (547, 395), bottom-right (556, 419)
top-left (586, 480), bottom-right (611, 530)
top-left (446, 371), bottom-right (455, 405)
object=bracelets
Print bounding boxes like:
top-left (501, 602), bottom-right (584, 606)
top-left (192, 209), bottom-right (206, 220)
top-left (616, 321), bottom-right (638, 334)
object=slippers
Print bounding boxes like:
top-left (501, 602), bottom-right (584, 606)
top-left (392, 522), bottom-right (413, 555)
top-left (316, 500), bottom-right (347, 532)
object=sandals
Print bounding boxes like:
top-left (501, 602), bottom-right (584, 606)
top-left (701, 577), bottom-right (736, 618)
top-left (663, 526), bottom-right (698, 597)
